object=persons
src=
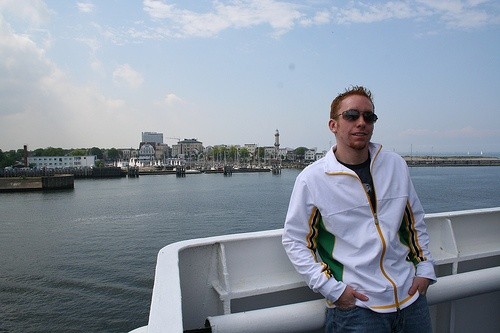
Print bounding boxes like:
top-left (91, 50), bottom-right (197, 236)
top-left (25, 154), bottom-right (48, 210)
top-left (282, 86), bottom-right (437, 333)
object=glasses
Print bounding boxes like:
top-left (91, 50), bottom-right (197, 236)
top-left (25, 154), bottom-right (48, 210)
top-left (332, 109), bottom-right (378, 123)
